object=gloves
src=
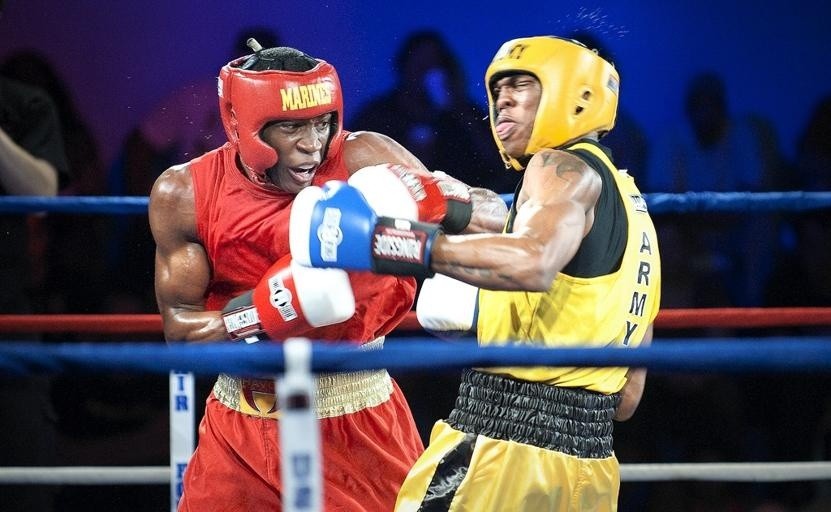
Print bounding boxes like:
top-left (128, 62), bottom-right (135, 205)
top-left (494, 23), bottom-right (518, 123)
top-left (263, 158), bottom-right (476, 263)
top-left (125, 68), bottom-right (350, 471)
top-left (222, 251), bottom-right (357, 350)
top-left (289, 180), bottom-right (445, 279)
top-left (346, 164), bottom-right (473, 234)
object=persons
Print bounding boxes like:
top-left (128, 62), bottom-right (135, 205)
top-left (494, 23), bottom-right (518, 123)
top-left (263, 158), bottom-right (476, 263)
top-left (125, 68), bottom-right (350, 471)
top-left (347, 31), bottom-right (524, 195)
top-left (573, 31), bottom-right (655, 195)
top-left (1, 128), bottom-right (57, 215)
top-left (683, 76), bottom-right (831, 191)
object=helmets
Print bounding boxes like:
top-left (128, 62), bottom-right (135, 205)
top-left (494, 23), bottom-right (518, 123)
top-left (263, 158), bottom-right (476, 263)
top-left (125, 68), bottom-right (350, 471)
top-left (484, 35), bottom-right (620, 172)
top-left (217, 38), bottom-right (343, 185)
